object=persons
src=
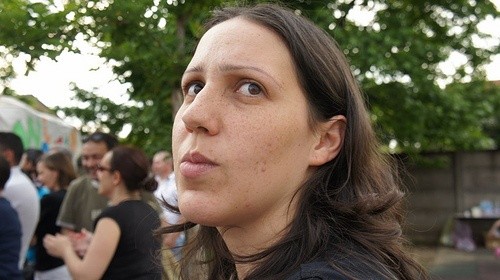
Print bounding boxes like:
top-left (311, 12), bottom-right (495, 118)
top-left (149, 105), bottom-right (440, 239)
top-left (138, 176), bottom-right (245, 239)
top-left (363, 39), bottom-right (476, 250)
top-left (148, 151), bottom-right (187, 265)
top-left (0, 157), bottom-right (22, 280)
top-left (24, 145), bottom-right (72, 267)
top-left (0, 132), bottom-right (40, 280)
top-left (43, 146), bottom-right (162, 280)
top-left (56, 132), bottom-right (168, 261)
top-left (152, 2), bottom-right (433, 280)
top-left (19, 148), bottom-right (87, 189)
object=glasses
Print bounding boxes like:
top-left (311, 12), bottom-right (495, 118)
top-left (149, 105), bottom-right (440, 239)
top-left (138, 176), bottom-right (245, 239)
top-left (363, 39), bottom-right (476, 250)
top-left (97, 165), bottom-right (111, 172)
top-left (81, 133), bottom-right (103, 142)
